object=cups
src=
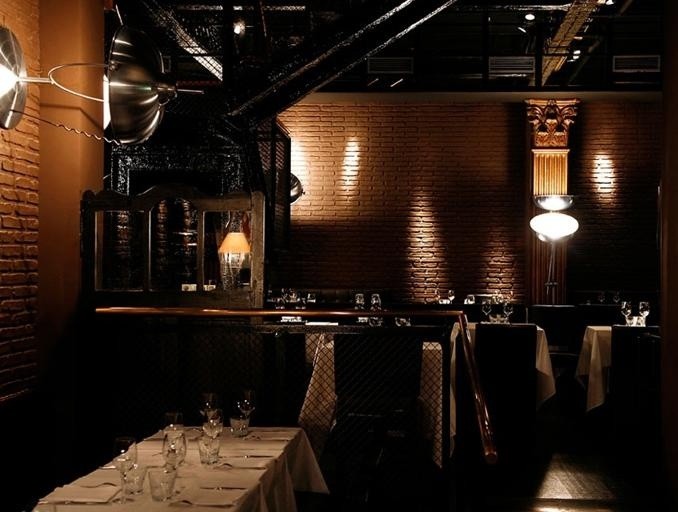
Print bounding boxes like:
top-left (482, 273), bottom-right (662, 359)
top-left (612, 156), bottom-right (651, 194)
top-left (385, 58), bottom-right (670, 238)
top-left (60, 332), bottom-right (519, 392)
top-left (356, 294), bottom-right (381, 306)
top-left (288, 290), bottom-right (298, 303)
top-left (129, 393), bottom-right (256, 501)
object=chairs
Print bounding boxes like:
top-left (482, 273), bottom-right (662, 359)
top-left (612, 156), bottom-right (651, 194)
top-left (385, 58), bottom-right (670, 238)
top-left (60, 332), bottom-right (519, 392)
top-left (241, 326), bottom-right (611, 484)
top-left (595, 327), bottom-right (664, 507)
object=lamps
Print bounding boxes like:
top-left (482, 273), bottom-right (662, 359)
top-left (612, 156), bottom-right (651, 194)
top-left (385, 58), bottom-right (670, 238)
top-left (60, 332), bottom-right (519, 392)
top-left (529, 194), bottom-right (579, 304)
top-left (218, 231), bottom-right (250, 291)
top-left (0, 24), bottom-right (205, 143)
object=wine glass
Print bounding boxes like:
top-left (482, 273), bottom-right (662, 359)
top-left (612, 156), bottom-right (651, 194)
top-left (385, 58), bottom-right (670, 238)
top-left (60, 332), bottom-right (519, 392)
top-left (621, 302), bottom-right (650, 328)
top-left (112, 440), bottom-right (138, 504)
top-left (448, 289), bottom-right (514, 325)
